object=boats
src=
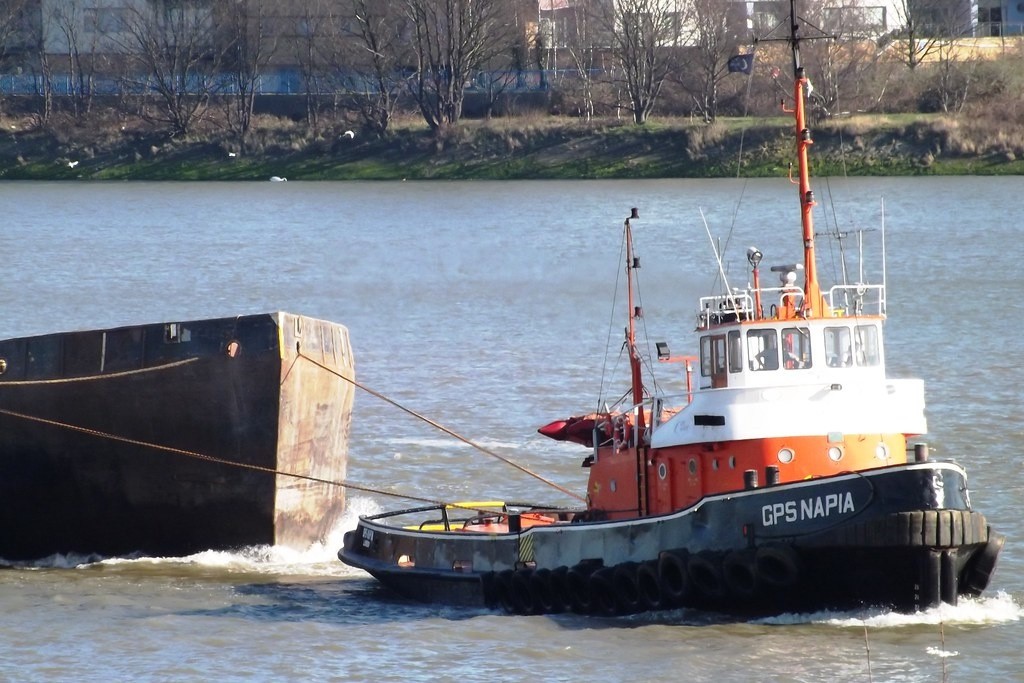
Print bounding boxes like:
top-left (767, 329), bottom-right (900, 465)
top-left (337, 0), bottom-right (1007, 617)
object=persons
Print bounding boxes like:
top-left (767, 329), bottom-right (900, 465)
top-left (845, 343), bottom-right (865, 363)
top-left (755, 337), bottom-right (799, 369)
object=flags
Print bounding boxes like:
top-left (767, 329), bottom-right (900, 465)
top-left (724, 54), bottom-right (755, 75)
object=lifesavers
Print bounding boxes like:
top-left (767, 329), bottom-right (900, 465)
top-left (613, 414), bottom-right (631, 449)
